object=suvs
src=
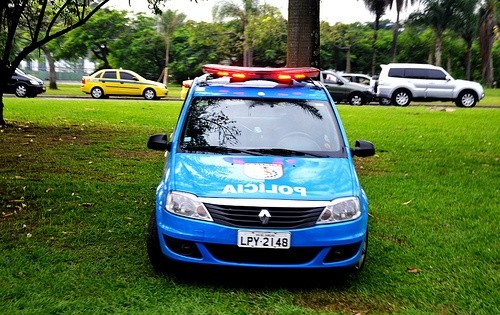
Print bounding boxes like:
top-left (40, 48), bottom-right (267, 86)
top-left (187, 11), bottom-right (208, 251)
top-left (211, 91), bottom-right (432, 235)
top-left (377, 63), bottom-right (485, 107)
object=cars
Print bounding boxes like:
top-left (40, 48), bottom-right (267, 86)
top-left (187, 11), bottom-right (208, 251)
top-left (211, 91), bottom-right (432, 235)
top-left (145, 62), bottom-right (375, 281)
top-left (2, 67), bottom-right (46, 98)
top-left (81, 68), bottom-right (168, 100)
